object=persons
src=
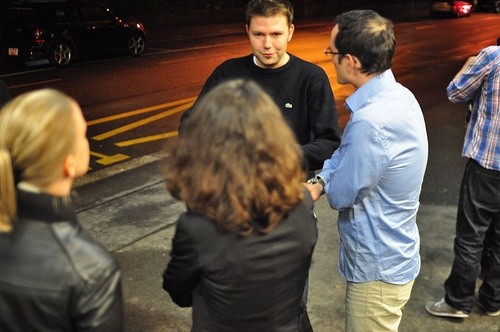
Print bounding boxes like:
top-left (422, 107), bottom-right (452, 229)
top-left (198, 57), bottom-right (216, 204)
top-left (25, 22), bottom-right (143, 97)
top-left (425, 36), bottom-right (500, 318)
top-left (160, 79), bottom-right (319, 332)
top-left (299, 10), bottom-right (429, 332)
top-left (0, 89), bottom-right (124, 332)
top-left (181, 0), bottom-right (343, 184)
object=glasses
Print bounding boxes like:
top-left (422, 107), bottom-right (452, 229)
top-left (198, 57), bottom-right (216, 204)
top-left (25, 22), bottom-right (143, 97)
top-left (324, 47), bottom-right (347, 56)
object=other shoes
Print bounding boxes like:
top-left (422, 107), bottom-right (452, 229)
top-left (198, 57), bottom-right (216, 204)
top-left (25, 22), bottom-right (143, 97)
top-left (486, 307), bottom-right (500, 316)
top-left (426, 297), bottom-right (470, 318)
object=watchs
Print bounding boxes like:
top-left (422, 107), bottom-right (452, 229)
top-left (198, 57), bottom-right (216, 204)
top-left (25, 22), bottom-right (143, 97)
top-left (307, 178), bottom-right (325, 196)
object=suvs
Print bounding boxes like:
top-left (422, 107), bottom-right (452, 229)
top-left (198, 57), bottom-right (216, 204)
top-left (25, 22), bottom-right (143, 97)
top-left (1, 0), bottom-right (148, 68)
